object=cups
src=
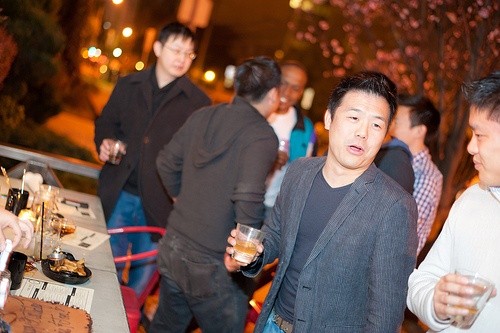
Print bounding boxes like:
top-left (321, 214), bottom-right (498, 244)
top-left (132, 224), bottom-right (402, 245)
top-left (232, 222), bottom-right (266, 265)
top-left (32, 191), bottom-right (56, 222)
top-left (447, 268), bottom-right (494, 329)
top-left (5, 188), bottom-right (29, 216)
top-left (35, 215), bottom-right (62, 259)
top-left (39, 184), bottom-right (61, 213)
top-left (6, 251), bottom-right (27, 290)
top-left (276, 139), bottom-right (289, 158)
top-left (106, 141), bottom-right (124, 165)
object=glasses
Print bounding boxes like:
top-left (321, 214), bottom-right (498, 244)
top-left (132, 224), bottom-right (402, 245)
top-left (163, 44), bottom-right (196, 60)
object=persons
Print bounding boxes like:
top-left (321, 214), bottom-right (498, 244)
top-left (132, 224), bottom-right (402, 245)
top-left (93, 22), bottom-right (212, 297)
top-left (262, 58), bottom-right (315, 215)
top-left (148, 55), bottom-right (279, 333)
top-left (375, 104), bottom-right (414, 194)
top-left (406, 72), bottom-right (500, 333)
top-left (393, 93), bottom-right (443, 257)
top-left (223, 70), bottom-right (419, 333)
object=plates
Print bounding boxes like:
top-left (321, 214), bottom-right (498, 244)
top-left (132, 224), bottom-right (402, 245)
top-left (40, 251), bottom-right (93, 285)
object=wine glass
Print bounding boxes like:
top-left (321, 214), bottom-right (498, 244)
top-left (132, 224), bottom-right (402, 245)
top-left (46, 217), bottom-right (77, 261)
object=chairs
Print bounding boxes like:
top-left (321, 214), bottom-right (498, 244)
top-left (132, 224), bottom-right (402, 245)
top-left (110, 226), bottom-right (167, 333)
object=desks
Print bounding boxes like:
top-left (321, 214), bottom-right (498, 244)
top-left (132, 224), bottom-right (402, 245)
top-left (1, 175), bottom-right (131, 333)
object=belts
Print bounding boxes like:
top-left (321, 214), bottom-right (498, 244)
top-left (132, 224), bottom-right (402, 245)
top-left (273, 314), bottom-right (293, 333)
